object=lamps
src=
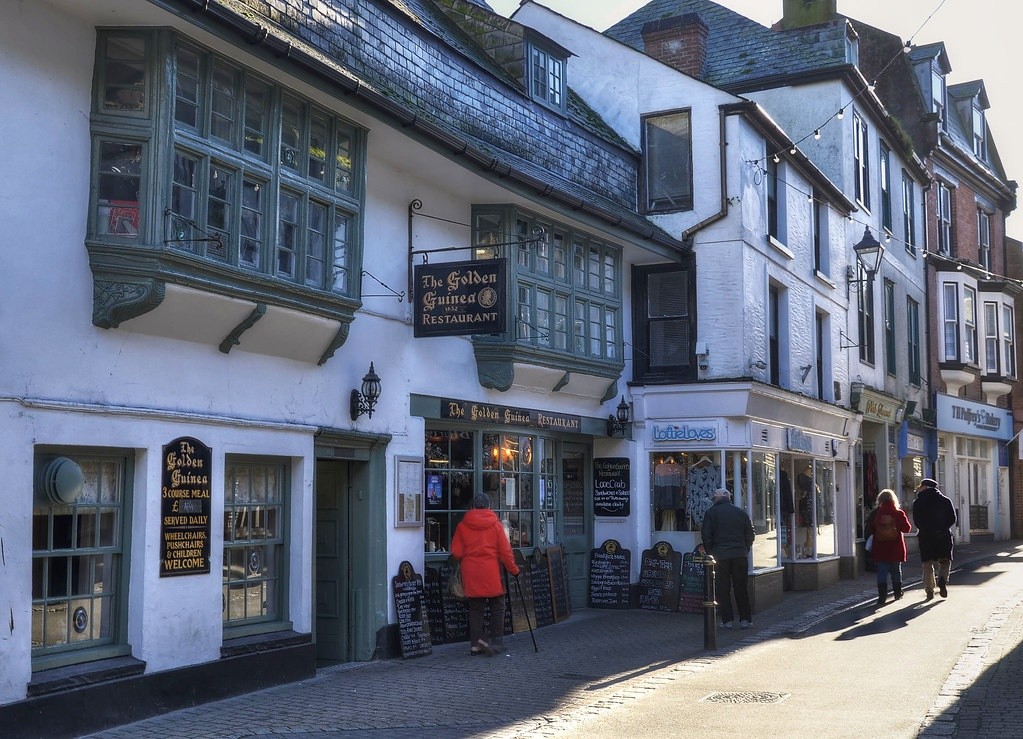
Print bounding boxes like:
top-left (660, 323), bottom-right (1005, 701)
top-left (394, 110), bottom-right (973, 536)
top-left (607, 394), bottom-right (630, 436)
top-left (846, 224), bottom-right (885, 301)
top-left (350, 361), bottom-right (382, 421)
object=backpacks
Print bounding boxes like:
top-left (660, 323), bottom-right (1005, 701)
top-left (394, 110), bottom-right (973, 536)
top-left (873, 510), bottom-right (898, 543)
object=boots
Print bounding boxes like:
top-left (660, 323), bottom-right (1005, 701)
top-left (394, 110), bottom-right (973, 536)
top-left (878, 582), bottom-right (887, 604)
top-left (892, 581), bottom-right (904, 600)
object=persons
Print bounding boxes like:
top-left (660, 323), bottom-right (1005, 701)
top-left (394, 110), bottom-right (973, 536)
top-left (701, 488), bottom-right (756, 629)
top-left (779, 460), bottom-right (794, 558)
top-left (451, 493), bottom-right (521, 656)
top-left (913, 478), bottom-right (956, 600)
top-left (796, 466), bottom-right (820, 557)
top-left (864, 489), bottom-right (912, 604)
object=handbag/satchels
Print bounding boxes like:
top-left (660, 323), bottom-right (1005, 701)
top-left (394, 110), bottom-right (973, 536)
top-left (865, 534), bottom-right (874, 551)
top-left (450, 561), bottom-right (465, 598)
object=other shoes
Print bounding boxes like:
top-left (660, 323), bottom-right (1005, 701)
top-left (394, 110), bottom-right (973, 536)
top-left (720, 620), bottom-right (733, 629)
top-left (936, 580), bottom-right (947, 597)
top-left (740, 620), bottom-right (755, 629)
top-left (489, 646), bottom-right (509, 654)
top-left (925, 589), bottom-right (934, 599)
top-left (471, 646), bottom-right (491, 656)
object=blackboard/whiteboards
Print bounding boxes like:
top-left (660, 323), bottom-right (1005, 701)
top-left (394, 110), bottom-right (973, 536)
top-left (563, 458), bottom-right (584, 515)
top-left (424, 567), bottom-right (446, 645)
top-left (438, 563), bottom-right (470, 644)
top-left (589, 539), bottom-right (631, 609)
top-left (979, 505), bottom-right (988, 529)
top-left (637, 541), bottom-right (682, 612)
top-left (393, 561), bottom-right (433, 660)
top-left (483, 562), bottom-right (513, 637)
top-left (507, 549), bottom-right (537, 633)
top-left (591, 457), bottom-right (631, 516)
top-left (545, 546), bottom-right (569, 624)
top-left (679, 543), bottom-right (719, 616)
top-left (969, 504), bottom-right (979, 529)
top-left (525, 547), bottom-right (554, 628)
top-left (559, 543), bottom-right (572, 616)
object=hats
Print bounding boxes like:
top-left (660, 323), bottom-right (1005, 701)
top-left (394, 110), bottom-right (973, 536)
top-left (709, 488), bottom-right (731, 502)
top-left (920, 478), bottom-right (938, 487)
